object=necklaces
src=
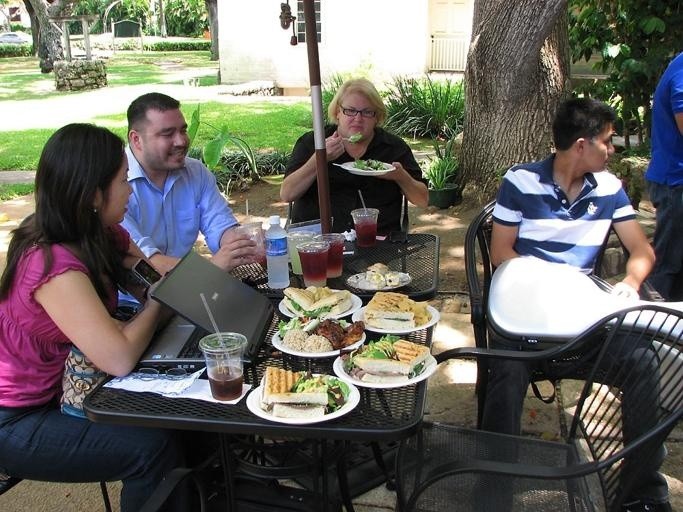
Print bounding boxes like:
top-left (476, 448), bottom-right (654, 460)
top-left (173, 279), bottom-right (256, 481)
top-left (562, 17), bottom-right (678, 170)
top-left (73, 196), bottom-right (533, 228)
top-left (343, 132), bottom-right (373, 161)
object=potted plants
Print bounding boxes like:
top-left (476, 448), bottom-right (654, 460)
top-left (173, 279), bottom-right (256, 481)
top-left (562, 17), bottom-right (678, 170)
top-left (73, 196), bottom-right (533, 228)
top-left (420, 154), bottom-right (464, 209)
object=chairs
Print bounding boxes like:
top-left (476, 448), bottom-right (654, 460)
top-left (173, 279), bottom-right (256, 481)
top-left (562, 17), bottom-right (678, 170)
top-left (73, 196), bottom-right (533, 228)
top-left (1, 433), bottom-right (215, 511)
top-left (393, 304), bottom-right (683, 512)
top-left (464, 199), bottom-right (668, 429)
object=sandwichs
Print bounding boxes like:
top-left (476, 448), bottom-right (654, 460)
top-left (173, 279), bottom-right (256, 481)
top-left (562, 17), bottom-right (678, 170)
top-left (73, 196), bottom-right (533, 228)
top-left (261, 393), bottom-right (327, 419)
top-left (284, 287), bottom-right (314, 315)
top-left (363, 292), bottom-right (429, 331)
top-left (262, 367), bottom-right (308, 412)
top-left (306, 289), bottom-right (352, 318)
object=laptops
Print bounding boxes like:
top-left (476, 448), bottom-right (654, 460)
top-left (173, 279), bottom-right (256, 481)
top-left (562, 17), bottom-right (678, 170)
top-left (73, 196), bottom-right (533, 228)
top-left (138, 249), bottom-right (275, 371)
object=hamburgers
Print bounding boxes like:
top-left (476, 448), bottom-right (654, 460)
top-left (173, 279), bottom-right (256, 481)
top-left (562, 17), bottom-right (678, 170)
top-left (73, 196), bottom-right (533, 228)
top-left (355, 336), bottom-right (431, 383)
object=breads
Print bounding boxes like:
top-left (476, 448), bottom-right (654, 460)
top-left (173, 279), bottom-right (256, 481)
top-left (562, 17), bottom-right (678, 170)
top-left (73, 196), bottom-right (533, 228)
top-left (366, 263), bottom-right (399, 287)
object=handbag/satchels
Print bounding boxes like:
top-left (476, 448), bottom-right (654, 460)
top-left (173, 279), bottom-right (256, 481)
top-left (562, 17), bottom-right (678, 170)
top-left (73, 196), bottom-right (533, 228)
top-left (58, 302), bottom-right (140, 419)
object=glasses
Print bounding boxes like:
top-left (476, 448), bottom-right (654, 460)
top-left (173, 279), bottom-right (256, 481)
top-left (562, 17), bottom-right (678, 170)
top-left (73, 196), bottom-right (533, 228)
top-left (133, 367), bottom-right (188, 380)
top-left (339, 104), bottom-right (376, 118)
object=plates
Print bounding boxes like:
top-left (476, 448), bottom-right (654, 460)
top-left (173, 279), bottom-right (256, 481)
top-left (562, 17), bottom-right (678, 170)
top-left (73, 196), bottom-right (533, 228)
top-left (246, 271), bottom-right (440, 425)
top-left (341, 161), bottom-right (397, 177)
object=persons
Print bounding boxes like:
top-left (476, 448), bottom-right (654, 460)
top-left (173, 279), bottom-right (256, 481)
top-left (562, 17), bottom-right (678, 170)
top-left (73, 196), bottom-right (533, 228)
top-left (646, 52), bottom-right (683, 303)
top-left (476, 98), bottom-right (673, 512)
top-left (0, 123), bottom-right (210, 511)
top-left (114, 93), bottom-right (267, 312)
top-left (280, 79), bottom-right (430, 273)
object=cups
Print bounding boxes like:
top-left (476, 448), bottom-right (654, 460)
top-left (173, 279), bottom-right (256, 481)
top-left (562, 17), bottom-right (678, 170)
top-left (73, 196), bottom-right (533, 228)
top-left (198, 333), bottom-right (247, 401)
top-left (236, 209), bottom-right (379, 288)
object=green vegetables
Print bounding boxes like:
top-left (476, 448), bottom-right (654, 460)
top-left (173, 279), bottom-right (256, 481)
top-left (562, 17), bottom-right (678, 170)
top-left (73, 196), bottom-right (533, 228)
top-left (278, 318), bottom-right (305, 336)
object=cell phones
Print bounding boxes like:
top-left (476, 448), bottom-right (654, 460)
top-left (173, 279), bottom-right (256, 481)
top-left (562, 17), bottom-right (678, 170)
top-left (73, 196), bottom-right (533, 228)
top-left (130, 259), bottom-right (163, 288)
